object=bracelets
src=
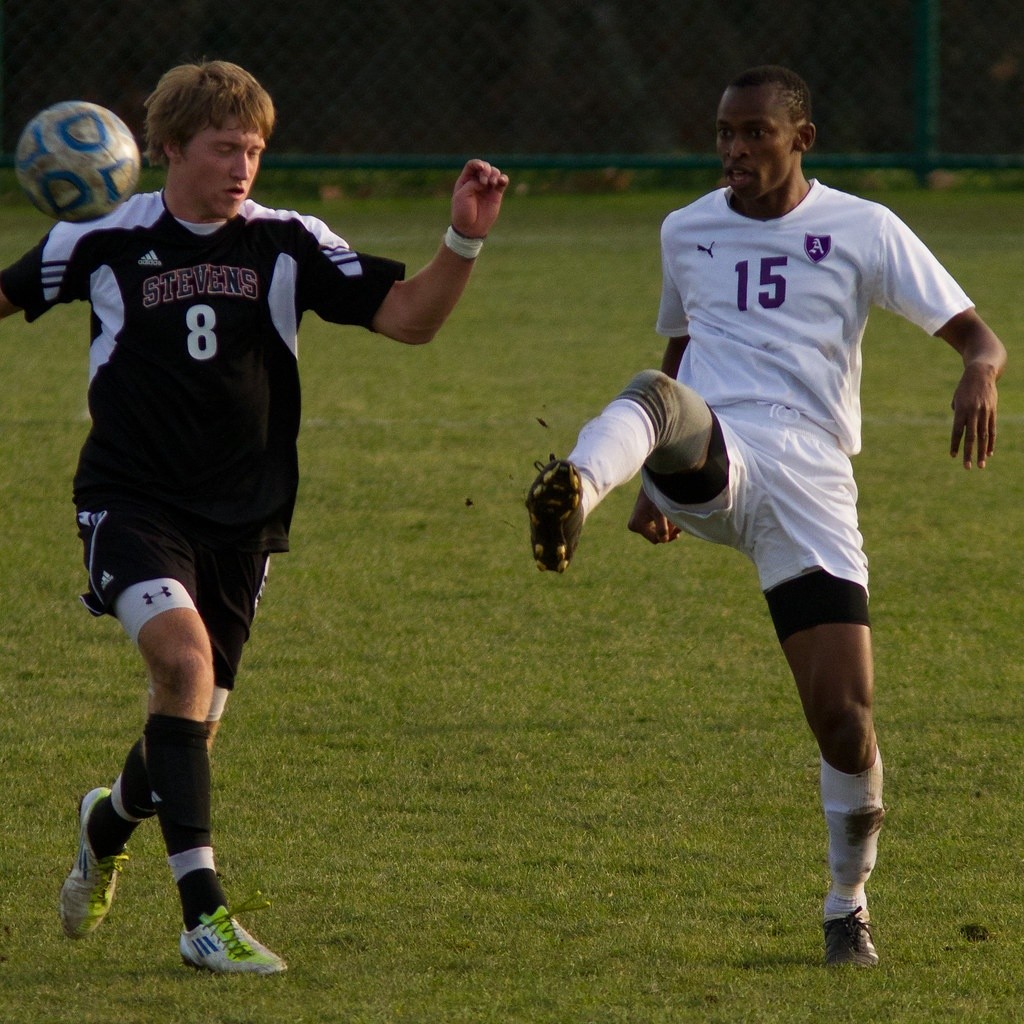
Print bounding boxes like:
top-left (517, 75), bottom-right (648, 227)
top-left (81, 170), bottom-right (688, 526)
top-left (443, 224), bottom-right (487, 259)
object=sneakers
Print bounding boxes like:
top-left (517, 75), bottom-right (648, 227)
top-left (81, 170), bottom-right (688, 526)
top-left (60, 787), bottom-right (128, 939)
top-left (823, 904), bottom-right (879, 969)
top-left (179, 889), bottom-right (287, 977)
top-left (525, 453), bottom-right (583, 575)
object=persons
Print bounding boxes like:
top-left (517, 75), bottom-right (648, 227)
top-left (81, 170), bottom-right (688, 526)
top-left (0, 61), bottom-right (508, 968)
top-left (524, 65), bottom-right (1007, 967)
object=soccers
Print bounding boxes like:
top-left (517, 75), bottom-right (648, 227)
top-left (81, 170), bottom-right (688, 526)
top-left (15, 101), bottom-right (140, 224)
